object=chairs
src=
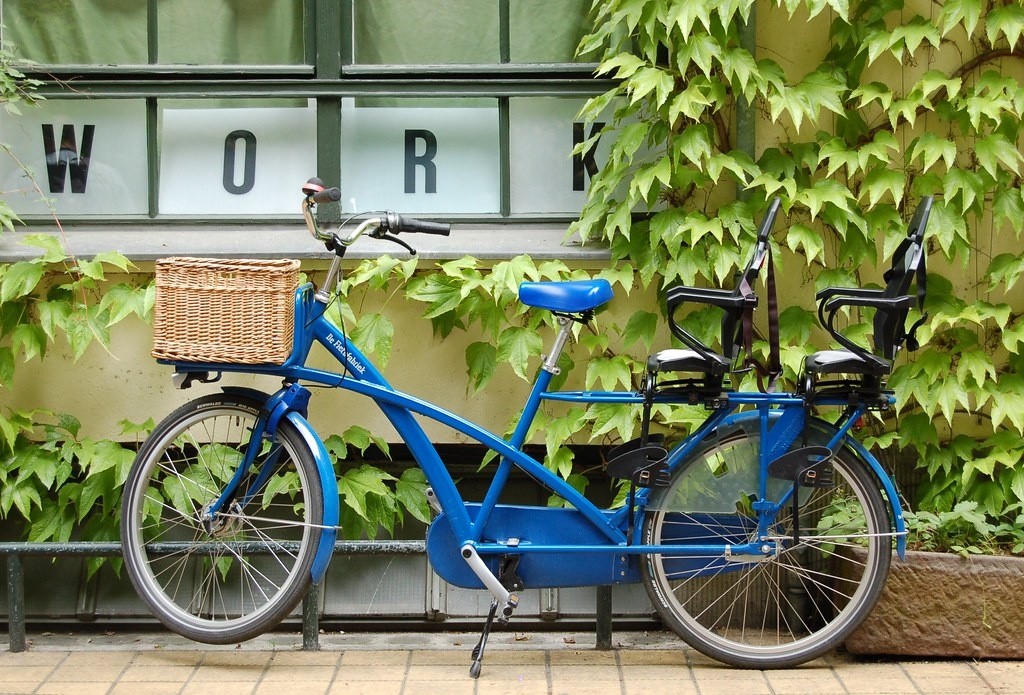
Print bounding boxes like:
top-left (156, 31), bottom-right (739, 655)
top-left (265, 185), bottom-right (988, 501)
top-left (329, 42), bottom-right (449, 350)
top-left (645, 197), bottom-right (782, 374)
top-left (802, 194), bottom-right (934, 373)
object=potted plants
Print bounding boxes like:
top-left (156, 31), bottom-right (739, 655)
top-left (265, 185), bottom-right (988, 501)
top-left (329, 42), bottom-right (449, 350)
top-left (815, 494), bottom-right (1024, 662)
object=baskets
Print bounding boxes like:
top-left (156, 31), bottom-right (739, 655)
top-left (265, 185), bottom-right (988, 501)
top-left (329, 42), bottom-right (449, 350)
top-left (151, 256), bottom-right (301, 366)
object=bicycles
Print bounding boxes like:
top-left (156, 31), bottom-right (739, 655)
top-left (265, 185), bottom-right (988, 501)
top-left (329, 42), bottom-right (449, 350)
top-left (120, 177), bottom-right (936, 680)
